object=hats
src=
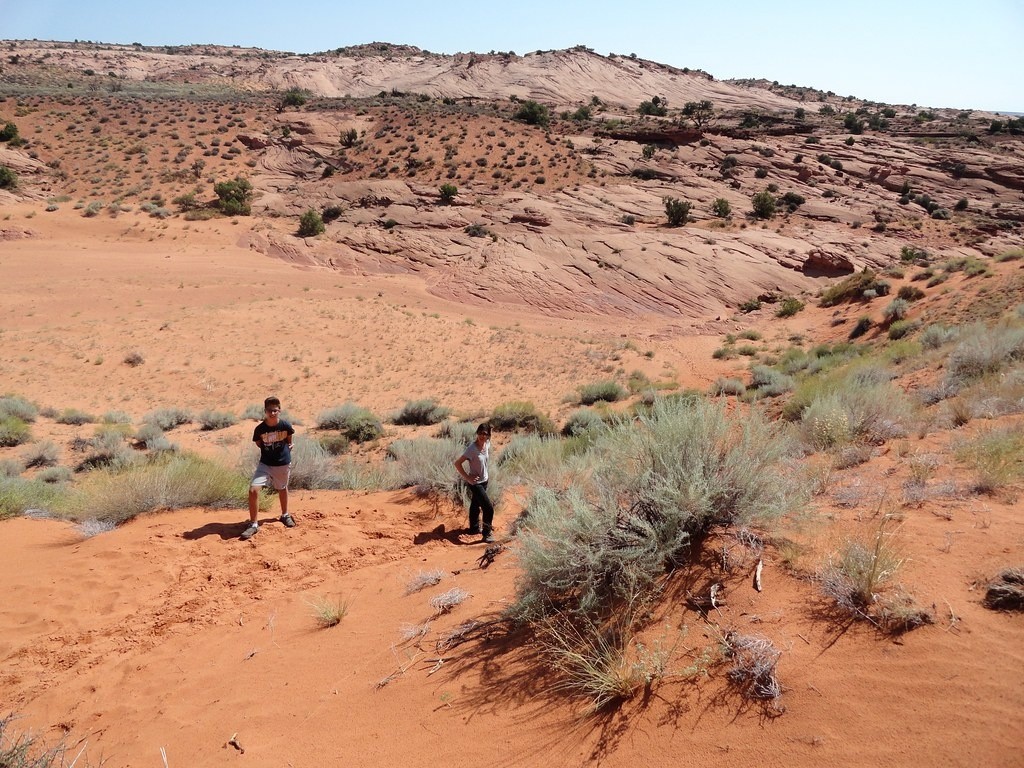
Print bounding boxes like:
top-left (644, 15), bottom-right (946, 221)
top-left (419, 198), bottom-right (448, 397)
top-left (264, 396), bottom-right (280, 406)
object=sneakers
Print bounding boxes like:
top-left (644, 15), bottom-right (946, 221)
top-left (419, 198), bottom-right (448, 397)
top-left (280, 514), bottom-right (295, 526)
top-left (241, 524), bottom-right (258, 538)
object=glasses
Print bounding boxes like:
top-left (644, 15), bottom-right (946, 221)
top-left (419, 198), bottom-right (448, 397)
top-left (266, 407), bottom-right (280, 413)
top-left (477, 431), bottom-right (488, 436)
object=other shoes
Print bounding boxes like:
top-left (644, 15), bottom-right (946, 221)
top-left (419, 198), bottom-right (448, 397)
top-left (478, 529), bottom-right (483, 533)
top-left (481, 534), bottom-right (494, 542)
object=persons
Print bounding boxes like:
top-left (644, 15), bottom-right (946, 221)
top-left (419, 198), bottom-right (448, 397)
top-left (240, 395), bottom-right (296, 540)
top-left (453, 421), bottom-right (498, 543)
